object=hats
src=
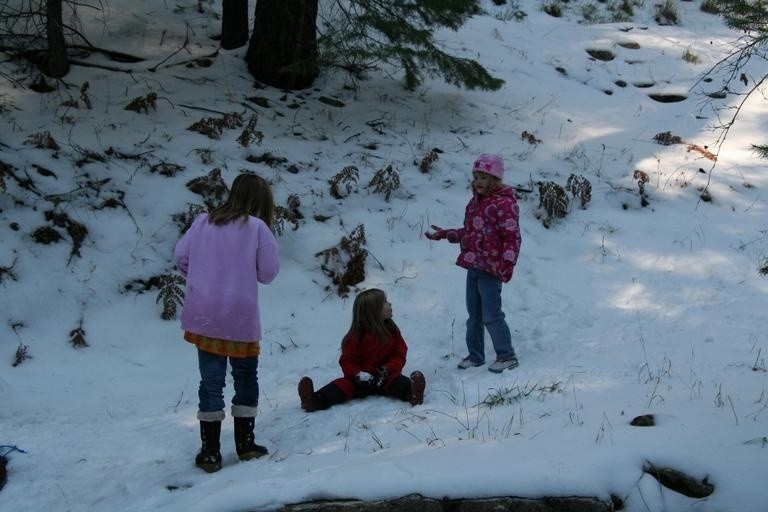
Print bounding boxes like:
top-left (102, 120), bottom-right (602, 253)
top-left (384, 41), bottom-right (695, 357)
top-left (471, 153), bottom-right (504, 181)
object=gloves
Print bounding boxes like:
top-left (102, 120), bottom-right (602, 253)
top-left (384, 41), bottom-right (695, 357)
top-left (497, 262), bottom-right (514, 283)
top-left (353, 366), bottom-right (389, 388)
top-left (425, 225), bottom-right (448, 240)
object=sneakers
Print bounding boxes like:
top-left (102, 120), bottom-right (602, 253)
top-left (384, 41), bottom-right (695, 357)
top-left (458, 356), bottom-right (486, 369)
top-left (488, 357), bottom-right (518, 372)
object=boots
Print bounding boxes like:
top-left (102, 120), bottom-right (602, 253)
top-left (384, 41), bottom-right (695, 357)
top-left (298, 377), bottom-right (346, 412)
top-left (195, 410), bottom-right (226, 473)
top-left (231, 404), bottom-right (267, 460)
top-left (389, 371), bottom-right (426, 405)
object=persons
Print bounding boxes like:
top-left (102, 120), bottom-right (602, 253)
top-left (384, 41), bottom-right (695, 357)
top-left (173, 173), bottom-right (280, 472)
top-left (425, 153), bottom-right (521, 372)
top-left (298, 288), bottom-right (425, 412)
